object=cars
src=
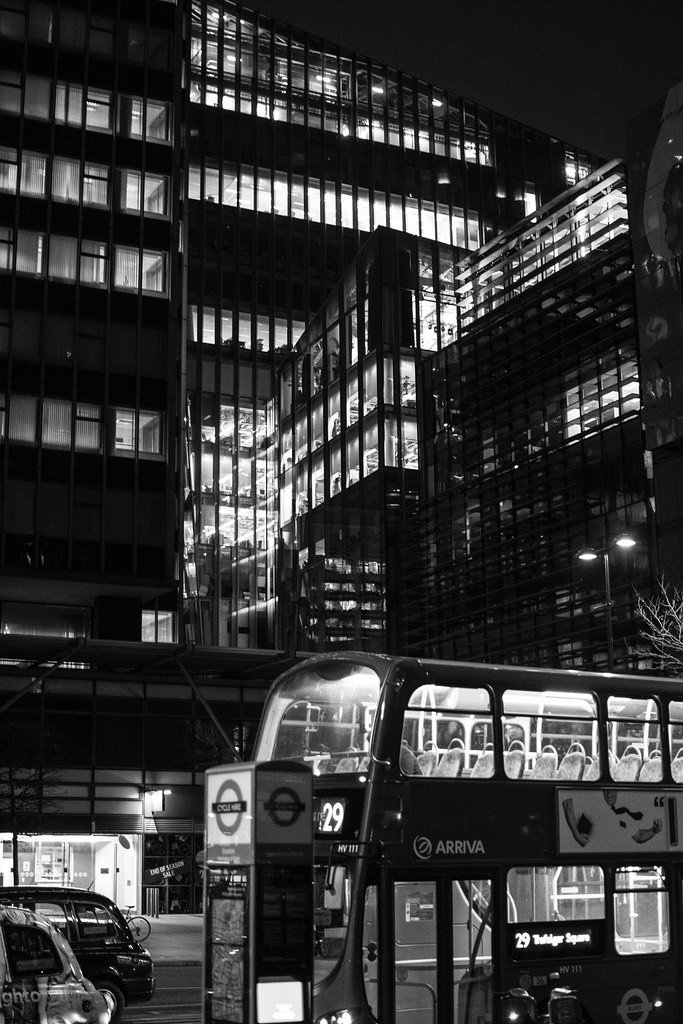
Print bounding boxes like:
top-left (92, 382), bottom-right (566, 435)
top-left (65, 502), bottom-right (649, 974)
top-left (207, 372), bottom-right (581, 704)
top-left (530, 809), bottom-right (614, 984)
top-left (0, 905), bottom-right (116, 1024)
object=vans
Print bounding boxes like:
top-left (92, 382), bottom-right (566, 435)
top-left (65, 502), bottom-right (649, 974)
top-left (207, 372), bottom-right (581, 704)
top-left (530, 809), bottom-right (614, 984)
top-left (0, 884), bottom-right (156, 1024)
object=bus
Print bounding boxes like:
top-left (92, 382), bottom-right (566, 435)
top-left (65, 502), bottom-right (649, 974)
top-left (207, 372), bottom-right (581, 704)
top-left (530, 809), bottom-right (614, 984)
top-left (250, 648), bottom-right (683, 1024)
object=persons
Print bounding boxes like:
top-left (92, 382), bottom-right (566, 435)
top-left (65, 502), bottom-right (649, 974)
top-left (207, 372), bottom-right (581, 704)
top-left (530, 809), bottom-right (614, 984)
top-left (366, 732), bottom-right (422, 776)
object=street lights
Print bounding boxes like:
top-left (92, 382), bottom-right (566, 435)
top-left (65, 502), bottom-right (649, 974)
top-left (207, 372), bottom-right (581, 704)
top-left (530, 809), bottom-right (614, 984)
top-left (575, 530), bottom-right (642, 670)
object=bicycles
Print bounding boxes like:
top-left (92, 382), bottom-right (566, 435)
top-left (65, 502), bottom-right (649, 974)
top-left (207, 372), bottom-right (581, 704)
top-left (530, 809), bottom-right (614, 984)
top-left (120, 905), bottom-right (151, 943)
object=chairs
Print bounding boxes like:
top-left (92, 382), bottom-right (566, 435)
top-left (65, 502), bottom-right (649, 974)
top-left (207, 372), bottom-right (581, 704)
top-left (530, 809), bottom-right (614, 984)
top-left (295, 743), bottom-right (683, 783)
